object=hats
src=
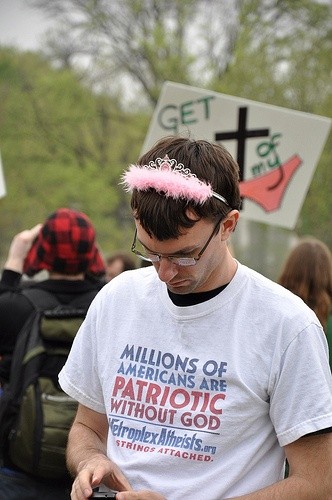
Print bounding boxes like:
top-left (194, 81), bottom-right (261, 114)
top-left (22, 208), bottom-right (107, 279)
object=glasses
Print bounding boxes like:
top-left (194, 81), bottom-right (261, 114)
top-left (131, 208), bottom-right (233, 266)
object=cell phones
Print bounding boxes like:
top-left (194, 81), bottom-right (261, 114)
top-left (87, 492), bottom-right (118, 500)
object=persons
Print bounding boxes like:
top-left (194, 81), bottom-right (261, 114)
top-left (276, 238), bottom-right (332, 369)
top-left (57, 137), bottom-right (332, 500)
top-left (0, 208), bottom-right (111, 500)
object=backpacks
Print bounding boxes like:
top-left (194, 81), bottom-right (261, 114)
top-left (0, 289), bottom-right (100, 478)
